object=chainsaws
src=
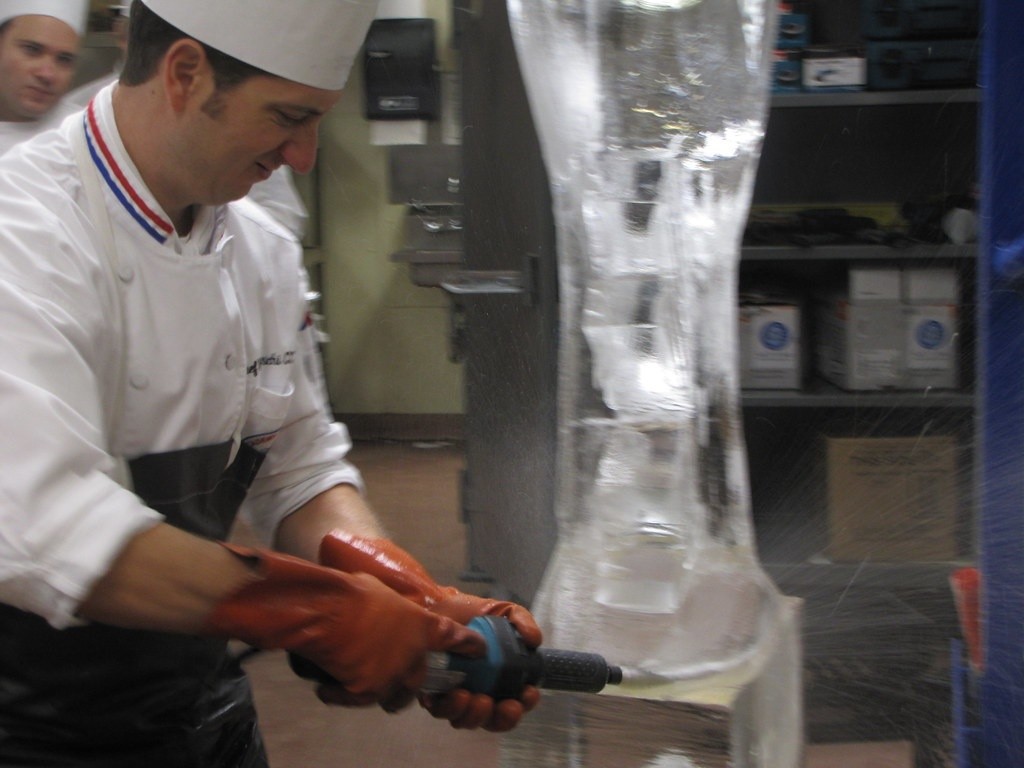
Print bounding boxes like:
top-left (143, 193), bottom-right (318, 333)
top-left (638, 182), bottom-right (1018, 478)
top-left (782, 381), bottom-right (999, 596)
top-left (414, 613), bottom-right (648, 706)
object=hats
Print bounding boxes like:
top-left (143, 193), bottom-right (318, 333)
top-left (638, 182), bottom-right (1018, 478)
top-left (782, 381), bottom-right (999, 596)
top-left (1, 0), bottom-right (92, 37)
top-left (139, 0), bottom-right (382, 94)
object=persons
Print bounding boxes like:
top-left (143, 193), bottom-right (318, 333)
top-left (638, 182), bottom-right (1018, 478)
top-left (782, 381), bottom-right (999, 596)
top-left (0, 0), bottom-right (131, 149)
top-left (0, 0), bottom-right (539, 768)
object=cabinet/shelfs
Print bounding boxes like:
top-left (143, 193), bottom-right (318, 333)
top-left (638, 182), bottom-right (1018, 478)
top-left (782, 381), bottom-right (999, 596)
top-left (734, 65), bottom-right (979, 600)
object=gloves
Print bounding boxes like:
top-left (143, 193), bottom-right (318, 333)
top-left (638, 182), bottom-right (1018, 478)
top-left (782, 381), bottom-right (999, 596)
top-left (196, 541), bottom-right (485, 717)
top-left (315, 532), bottom-right (542, 731)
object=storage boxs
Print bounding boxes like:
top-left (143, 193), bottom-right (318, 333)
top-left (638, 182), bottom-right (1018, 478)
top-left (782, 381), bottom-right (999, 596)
top-left (740, 259), bottom-right (963, 564)
